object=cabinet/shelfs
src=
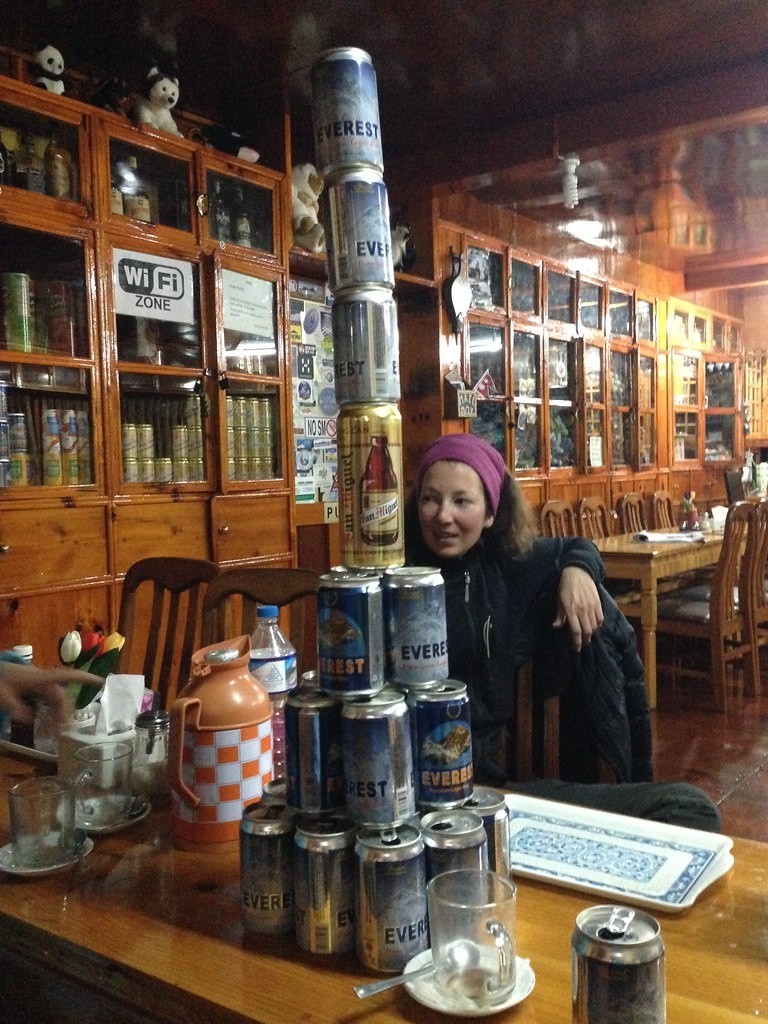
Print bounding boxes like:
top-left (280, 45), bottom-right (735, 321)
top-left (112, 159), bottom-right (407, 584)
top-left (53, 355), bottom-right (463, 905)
top-left (399, 189), bottom-right (668, 535)
top-left (666, 295), bottom-right (746, 521)
top-left (0, 46), bottom-right (298, 712)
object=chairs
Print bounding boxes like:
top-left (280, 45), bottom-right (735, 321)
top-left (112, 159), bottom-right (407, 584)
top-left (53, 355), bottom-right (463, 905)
top-left (724, 470), bottom-right (745, 505)
top-left (199, 568), bottom-right (323, 683)
top-left (118, 556), bottom-right (234, 711)
top-left (538, 490), bottom-right (768, 714)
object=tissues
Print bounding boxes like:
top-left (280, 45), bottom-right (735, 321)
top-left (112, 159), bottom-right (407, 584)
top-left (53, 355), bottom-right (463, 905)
top-left (56, 670), bottom-right (144, 787)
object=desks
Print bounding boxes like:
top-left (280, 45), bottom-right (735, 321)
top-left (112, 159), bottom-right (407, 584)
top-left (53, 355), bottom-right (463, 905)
top-left (0, 749), bottom-right (768, 1024)
top-left (591, 522), bottom-right (766, 710)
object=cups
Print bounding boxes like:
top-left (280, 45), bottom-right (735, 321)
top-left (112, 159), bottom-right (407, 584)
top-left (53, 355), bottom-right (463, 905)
top-left (72, 740), bottom-right (135, 824)
top-left (7, 775), bottom-right (75, 865)
top-left (427, 868), bottom-right (519, 1007)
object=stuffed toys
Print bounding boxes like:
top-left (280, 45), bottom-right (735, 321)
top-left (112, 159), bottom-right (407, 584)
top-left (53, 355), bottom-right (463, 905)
top-left (291, 163), bottom-right (329, 254)
top-left (132, 66), bottom-right (184, 138)
top-left (391, 222), bottom-right (411, 272)
top-left (29, 43), bottom-right (69, 96)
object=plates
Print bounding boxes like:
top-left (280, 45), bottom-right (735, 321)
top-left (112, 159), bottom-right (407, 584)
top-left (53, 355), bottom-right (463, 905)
top-left (403, 946), bottom-right (537, 1016)
top-left (0, 830), bottom-right (95, 875)
top-left (502, 792), bottom-right (733, 909)
top-left (56, 794), bottom-right (154, 837)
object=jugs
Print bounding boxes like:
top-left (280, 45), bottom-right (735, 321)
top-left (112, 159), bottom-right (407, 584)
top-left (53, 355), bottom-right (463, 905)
top-left (167, 635), bottom-right (277, 845)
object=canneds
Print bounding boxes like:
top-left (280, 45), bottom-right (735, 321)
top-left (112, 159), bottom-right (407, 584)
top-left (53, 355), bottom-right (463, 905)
top-left (0, 384), bottom-right (91, 486)
top-left (125, 396), bottom-right (275, 482)
top-left (311, 46), bottom-right (395, 293)
top-left (332, 286), bottom-right (401, 407)
top-left (238, 670), bottom-right (510, 973)
top-left (0, 272), bottom-right (77, 358)
top-left (336, 401), bottom-right (406, 572)
top-left (570, 904), bottom-right (667, 1024)
top-left (315, 564), bottom-right (449, 700)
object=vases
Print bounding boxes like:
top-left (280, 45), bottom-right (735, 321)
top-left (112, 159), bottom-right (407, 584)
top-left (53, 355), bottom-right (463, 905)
top-left (687, 509), bottom-right (699, 531)
top-left (33, 700), bottom-right (62, 755)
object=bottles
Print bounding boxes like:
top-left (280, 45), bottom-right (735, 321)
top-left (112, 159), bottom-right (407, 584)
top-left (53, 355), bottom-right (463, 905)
top-left (45, 128), bottom-right (71, 202)
top-left (131, 710), bottom-right (168, 809)
top-left (10, 643), bottom-right (33, 749)
top-left (249, 606), bottom-right (298, 775)
top-left (705, 443), bottom-right (716, 462)
top-left (687, 507), bottom-right (710, 530)
top-left (211, 180), bottom-right (251, 247)
top-left (359, 437), bottom-right (399, 544)
top-left (111, 154), bottom-right (152, 222)
top-left (0, 128), bottom-right (45, 192)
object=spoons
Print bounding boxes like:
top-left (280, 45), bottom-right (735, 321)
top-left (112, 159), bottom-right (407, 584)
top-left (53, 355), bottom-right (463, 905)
top-left (354, 940), bottom-right (479, 1002)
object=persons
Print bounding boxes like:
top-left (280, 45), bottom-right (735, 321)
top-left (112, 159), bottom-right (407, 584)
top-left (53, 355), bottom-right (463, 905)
top-left (404, 433), bottom-right (725, 836)
top-left (0, 650), bottom-right (106, 735)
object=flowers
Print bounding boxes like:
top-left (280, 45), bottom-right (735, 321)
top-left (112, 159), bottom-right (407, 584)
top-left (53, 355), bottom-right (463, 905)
top-left (681, 490), bottom-right (696, 516)
top-left (57, 630), bottom-right (126, 711)
